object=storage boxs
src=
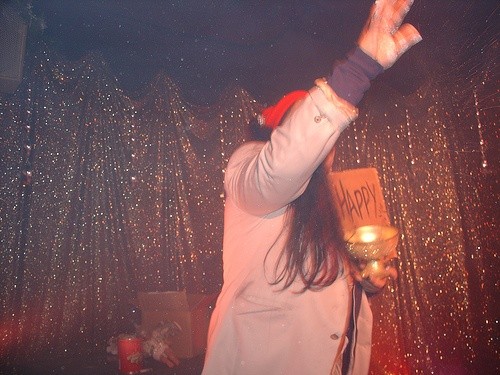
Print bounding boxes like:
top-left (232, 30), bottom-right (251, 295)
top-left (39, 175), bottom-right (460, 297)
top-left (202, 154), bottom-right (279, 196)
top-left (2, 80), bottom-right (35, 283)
top-left (137, 292), bottom-right (218, 361)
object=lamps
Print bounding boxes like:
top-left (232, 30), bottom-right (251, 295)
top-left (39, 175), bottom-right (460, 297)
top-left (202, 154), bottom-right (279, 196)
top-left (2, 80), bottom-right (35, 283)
top-left (343, 224), bottom-right (400, 280)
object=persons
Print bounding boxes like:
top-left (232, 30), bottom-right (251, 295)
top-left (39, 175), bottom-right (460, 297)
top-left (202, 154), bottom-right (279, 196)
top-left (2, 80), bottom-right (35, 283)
top-left (202, 0), bottom-right (423, 375)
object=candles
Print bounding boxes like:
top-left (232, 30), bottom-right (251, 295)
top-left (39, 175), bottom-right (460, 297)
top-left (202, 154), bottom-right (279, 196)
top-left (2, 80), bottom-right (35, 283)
top-left (117, 337), bottom-right (143, 375)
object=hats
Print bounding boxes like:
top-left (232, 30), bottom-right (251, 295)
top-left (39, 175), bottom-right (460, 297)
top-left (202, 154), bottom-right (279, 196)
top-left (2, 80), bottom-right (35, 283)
top-left (258, 87), bottom-right (307, 131)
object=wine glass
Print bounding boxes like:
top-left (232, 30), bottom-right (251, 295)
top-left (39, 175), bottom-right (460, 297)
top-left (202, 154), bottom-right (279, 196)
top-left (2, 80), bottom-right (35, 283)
top-left (344, 223), bottom-right (400, 276)
